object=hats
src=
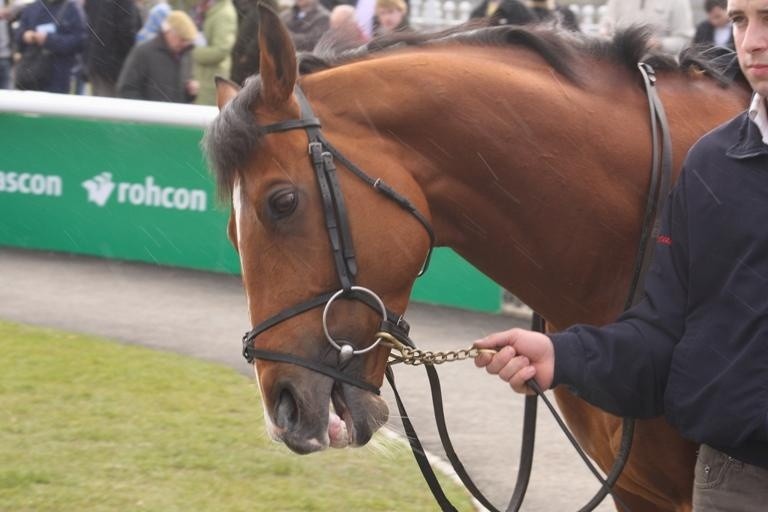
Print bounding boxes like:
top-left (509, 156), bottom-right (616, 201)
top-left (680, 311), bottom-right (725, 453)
top-left (166, 10), bottom-right (200, 41)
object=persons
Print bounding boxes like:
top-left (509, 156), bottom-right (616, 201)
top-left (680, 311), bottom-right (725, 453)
top-left (315, 5), bottom-right (368, 60)
top-left (373, 1), bottom-right (412, 38)
top-left (277, 1), bottom-right (331, 51)
top-left (118, 10), bottom-right (200, 104)
top-left (8, 1), bottom-right (90, 94)
top-left (190, 2), bottom-right (240, 106)
top-left (471, 1), bottom-right (767, 510)
top-left (70, 2), bottom-right (170, 96)
top-left (692, 0), bottom-right (738, 53)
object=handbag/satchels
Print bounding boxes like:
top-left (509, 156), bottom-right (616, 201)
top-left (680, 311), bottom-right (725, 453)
top-left (16, 67), bottom-right (42, 90)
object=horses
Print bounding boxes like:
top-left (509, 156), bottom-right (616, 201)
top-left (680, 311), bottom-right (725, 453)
top-left (200, 0), bottom-right (755, 511)
